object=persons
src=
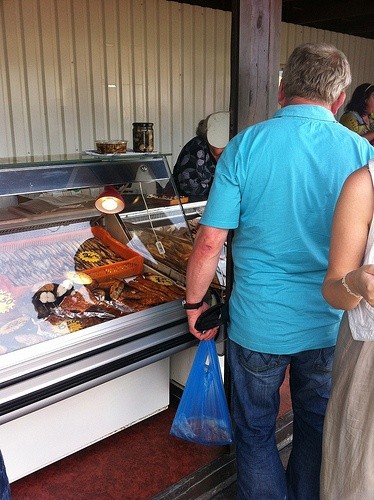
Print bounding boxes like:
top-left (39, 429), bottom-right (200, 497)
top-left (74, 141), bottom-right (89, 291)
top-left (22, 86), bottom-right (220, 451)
top-left (181, 43), bottom-right (374, 500)
top-left (320, 158), bottom-right (374, 500)
top-left (339, 83), bottom-right (374, 147)
top-left (161, 114), bottom-right (226, 195)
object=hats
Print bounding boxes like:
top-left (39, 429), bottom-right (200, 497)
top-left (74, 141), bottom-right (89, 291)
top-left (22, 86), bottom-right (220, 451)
top-left (207, 112), bottom-right (231, 149)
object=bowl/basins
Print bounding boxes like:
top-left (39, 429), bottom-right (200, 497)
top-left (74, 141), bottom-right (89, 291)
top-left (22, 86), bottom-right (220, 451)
top-left (96, 139), bottom-right (128, 155)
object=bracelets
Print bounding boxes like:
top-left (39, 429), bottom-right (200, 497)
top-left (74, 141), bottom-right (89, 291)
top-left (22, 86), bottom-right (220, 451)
top-left (342, 272), bottom-right (363, 299)
top-left (182, 297), bottom-right (203, 309)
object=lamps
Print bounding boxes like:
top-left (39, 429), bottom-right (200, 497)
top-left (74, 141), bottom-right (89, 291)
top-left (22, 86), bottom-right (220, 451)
top-left (94, 185), bottom-right (125, 215)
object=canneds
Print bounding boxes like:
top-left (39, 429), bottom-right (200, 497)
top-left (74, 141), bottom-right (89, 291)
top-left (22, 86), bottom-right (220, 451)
top-left (132, 122), bottom-right (154, 153)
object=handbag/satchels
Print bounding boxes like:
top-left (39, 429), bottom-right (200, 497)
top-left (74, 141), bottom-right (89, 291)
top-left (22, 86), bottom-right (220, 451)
top-left (169, 338), bottom-right (234, 445)
